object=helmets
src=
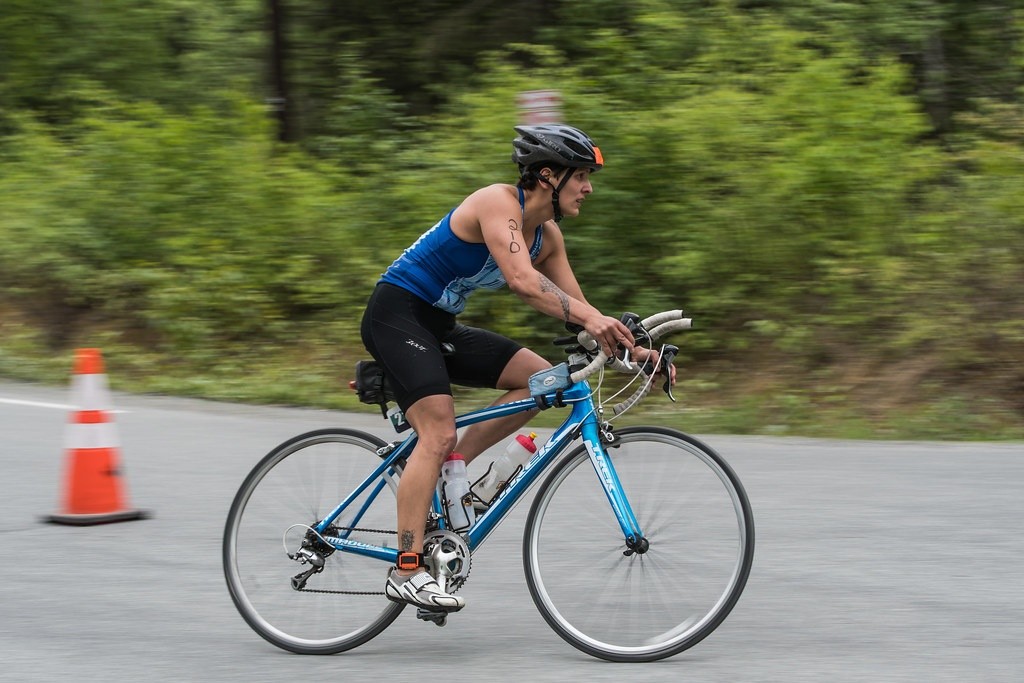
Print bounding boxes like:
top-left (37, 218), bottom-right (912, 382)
top-left (512, 122), bottom-right (606, 172)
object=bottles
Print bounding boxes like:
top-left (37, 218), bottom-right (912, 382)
top-left (472, 432), bottom-right (538, 503)
top-left (441, 450), bottom-right (475, 532)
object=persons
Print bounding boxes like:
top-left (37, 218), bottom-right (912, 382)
top-left (362, 122), bottom-right (676, 612)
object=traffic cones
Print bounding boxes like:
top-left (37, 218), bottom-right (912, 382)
top-left (36, 349), bottom-right (147, 525)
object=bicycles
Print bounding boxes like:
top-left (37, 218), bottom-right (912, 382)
top-left (222, 310), bottom-right (755, 664)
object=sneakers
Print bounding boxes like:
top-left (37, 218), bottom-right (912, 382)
top-left (384, 567), bottom-right (466, 612)
